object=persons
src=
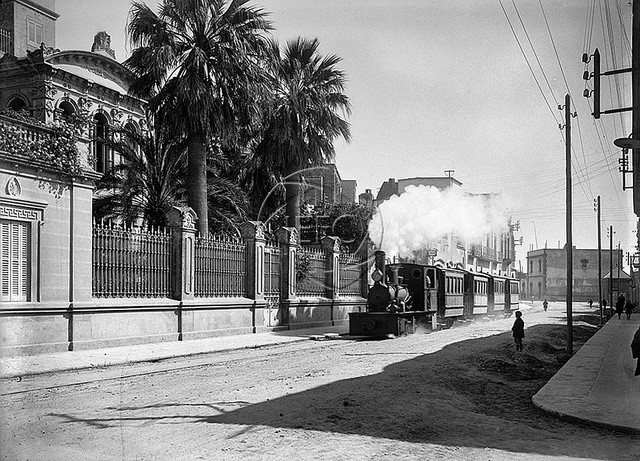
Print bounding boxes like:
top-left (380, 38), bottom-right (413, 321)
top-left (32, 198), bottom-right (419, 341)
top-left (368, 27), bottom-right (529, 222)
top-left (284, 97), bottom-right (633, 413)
top-left (512, 312), bottom-right (526, 351)
top-left (615, 295), bottom-right (625, 318)
top-left (588, 299), bottom-right (593, 308)
top-left (626, 299), bottom-right (632, 319)
top-left (543, 299), bottom-right (549, 308)
top-left (631, 326), bottom-right (640, 376)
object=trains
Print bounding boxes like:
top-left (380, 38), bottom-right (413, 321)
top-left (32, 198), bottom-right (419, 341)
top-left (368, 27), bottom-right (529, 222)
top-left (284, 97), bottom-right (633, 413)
top-left (348, 251), bottom-right (520, 338)
